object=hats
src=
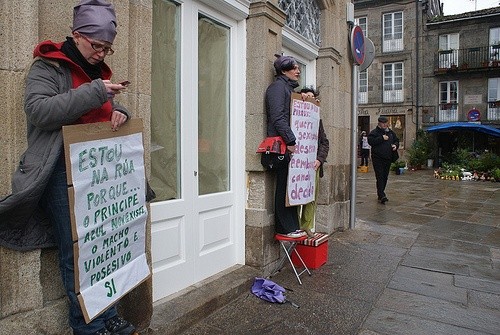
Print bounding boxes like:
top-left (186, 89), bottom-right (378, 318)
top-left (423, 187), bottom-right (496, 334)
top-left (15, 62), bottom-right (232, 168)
top-left (362, 131), bottom-right (366, 133)
top-left (378, 116), bottom-right (388, 123)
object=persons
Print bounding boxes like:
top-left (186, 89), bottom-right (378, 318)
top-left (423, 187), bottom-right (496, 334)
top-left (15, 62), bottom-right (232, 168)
top-left (359, 130), bottom-right (370, 166)
top-left (300, 89), bottom-right (329, 233)
top-left (0, 0), bottom-right (156, 335)
top-left (263, 55), bottom-right (310, 238)
top-left (368, 117), bottom-right (400, 202)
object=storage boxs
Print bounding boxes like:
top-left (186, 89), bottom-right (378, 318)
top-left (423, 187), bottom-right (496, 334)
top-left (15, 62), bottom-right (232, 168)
top-left (291, 233), bottom-right (329, 270)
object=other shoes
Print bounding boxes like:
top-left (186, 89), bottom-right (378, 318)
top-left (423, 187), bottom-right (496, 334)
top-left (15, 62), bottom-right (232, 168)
top-left (277, 229), bottom-right (307, 238)
top-left (357, 166), bottom-right (362, 168)
top-left (378, 197), bottom-right (388, 205)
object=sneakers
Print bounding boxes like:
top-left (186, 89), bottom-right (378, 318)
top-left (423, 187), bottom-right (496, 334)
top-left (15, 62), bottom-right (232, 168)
top-left (73, 326), bottom-right (120, 335)
top-left (105, 314), bottom-right (136, 335)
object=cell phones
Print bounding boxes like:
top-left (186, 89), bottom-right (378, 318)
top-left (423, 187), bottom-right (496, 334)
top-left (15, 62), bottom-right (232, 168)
top-left (116, 80), bottom-right (131, 86)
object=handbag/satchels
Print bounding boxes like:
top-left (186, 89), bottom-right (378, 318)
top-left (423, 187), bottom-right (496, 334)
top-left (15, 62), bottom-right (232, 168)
top-left (392, 150), bottom-right (399, 163)
top-left (255, 135), bottom-right (290, 170)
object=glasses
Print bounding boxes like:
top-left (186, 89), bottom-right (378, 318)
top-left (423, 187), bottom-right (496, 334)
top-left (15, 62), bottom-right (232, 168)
top-left (80, 33), bottom-right (114, 55)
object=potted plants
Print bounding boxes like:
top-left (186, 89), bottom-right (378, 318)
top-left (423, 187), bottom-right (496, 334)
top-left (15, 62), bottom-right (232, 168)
top-left (461, 62), bottom-right (468, 69)
top-left (480, 58), bottom-right (500, 67)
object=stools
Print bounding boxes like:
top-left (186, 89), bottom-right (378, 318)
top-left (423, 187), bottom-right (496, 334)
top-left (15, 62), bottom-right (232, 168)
top-left (270, 234), bottom-right (312, 285)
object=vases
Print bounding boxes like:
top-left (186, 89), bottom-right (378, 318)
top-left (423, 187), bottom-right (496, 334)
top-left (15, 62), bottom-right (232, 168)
top-left (439, 67), bottom-right (447, 72)
top-left (451, 65), bottom-right (457, 69)
